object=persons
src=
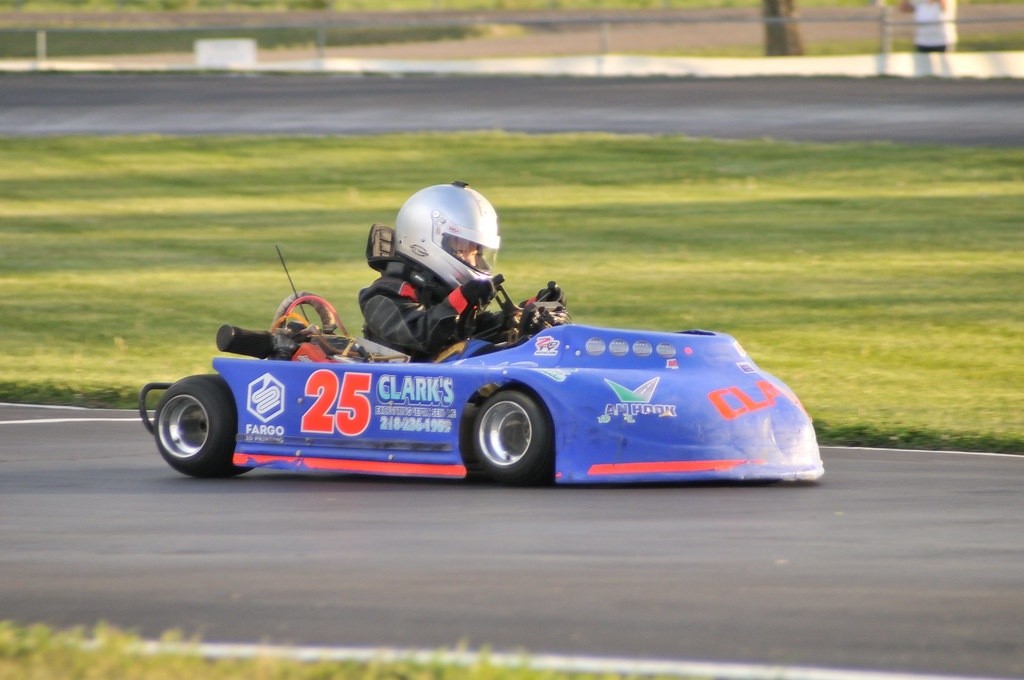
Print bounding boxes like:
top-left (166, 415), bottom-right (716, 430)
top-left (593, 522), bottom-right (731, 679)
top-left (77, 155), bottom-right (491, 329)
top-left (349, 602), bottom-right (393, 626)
top-left (899, 0), bottom-right (958, 52)
top-left (358, 182), bottom-right (573, 366)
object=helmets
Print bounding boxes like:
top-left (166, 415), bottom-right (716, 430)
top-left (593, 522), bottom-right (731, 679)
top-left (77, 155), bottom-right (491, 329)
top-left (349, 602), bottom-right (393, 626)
top-left (394, 182), bottom-right (501, 294)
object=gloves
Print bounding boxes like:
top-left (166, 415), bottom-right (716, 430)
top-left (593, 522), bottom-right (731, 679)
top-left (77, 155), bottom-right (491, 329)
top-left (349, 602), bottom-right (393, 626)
top-left (519, 288), bottom-right (567, 307)
top-left (448, 280), bottom-right (495, 314)
